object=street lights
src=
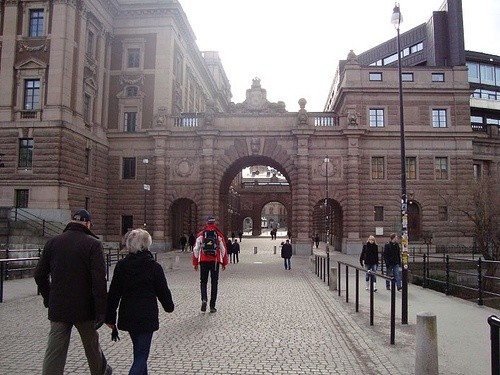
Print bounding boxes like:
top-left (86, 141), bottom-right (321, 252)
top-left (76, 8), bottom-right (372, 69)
top-left (324, 155), bottom-right (330, 286)
top-left (391, 3), bottom-right (410, 326)
top-left (142, 155), bottom-right (150, 231)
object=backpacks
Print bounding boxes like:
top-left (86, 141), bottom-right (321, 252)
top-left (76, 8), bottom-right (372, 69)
top-left (199, 230), bottom-right (219, 256)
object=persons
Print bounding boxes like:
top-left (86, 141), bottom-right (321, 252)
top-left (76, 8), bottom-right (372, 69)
top-left (287, 230), bottom-right (292, 239)
top-left (106, 227), bottom-right (175, 375)
top-left (315, 234), bottom-right (320, 248)
top-left (281, 240), bottom-right (292, 270)
top-left (232, 239), bottom-right (240, 264)
top-left (179, 235), bottom-right (187, 251)
top-left (238, 229), bottom-right (243, 242)
top-left (188, 233), bottom-right (196, 253)
top-left (231, 232), bottom-right (235, 242)
top-left (383, 233), bottom-right (402, 291)
top-left (34, 208), bottom-right (113, 375)
top-left (192, 214), bottom-right (229, 315)
top-left (226, 240), bottom-right (233, 263)
top-left (271, 227), bottom-right (277, 240)
top-left (360, 234), bottom-right (379, 292)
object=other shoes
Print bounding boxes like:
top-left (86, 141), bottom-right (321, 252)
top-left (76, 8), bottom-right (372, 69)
top-left (210, 306), bottom-right (217, 313)
top-left (387, 285), bottom-right (390, 290)
top-left (201, 300), bottom-right (206, 311)
top-left (397, 287), bottom-right (402, 291)
top-left (105, 365), bottom-right (112, 375)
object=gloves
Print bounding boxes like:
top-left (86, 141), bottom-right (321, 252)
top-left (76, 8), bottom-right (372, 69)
top-left (111, 325), bottom-right (120, 342)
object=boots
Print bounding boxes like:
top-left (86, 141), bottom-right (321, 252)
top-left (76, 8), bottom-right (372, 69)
top-left (365, 281), bottom-right (369, 290)
top-left (373, 282), bottom-right (377, 292)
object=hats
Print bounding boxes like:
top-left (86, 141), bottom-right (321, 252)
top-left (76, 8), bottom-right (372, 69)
top-left (72, 208), bottom-right (92, 228)
top-left (206, 216), bottom-right (216, 222)
